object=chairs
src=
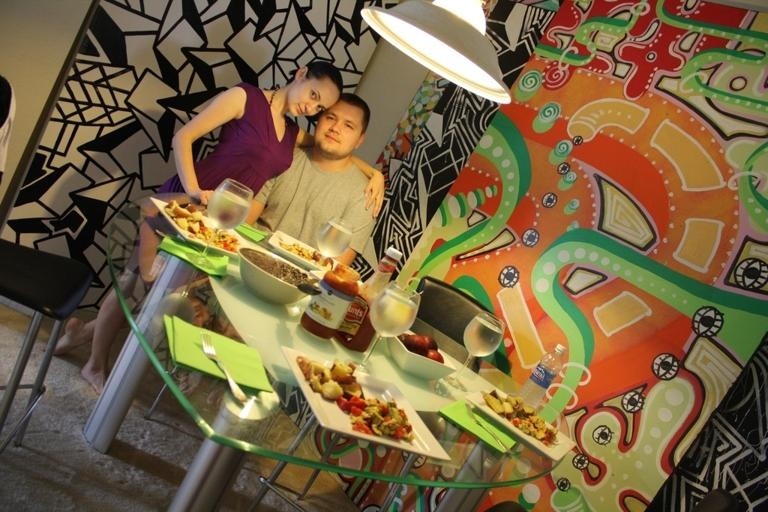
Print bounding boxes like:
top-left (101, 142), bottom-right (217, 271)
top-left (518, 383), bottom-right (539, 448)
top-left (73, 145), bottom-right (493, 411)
top-left (0, 74), bottom-right (95, 461)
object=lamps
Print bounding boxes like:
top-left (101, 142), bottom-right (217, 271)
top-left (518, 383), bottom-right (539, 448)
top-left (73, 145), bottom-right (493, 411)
top-left (359, 0), bottom-right (513, 106)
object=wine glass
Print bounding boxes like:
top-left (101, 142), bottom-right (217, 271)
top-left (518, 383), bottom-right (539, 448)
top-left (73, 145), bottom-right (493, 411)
top-left (442, 310), bottom-right (505, 392)
top-left (346, 278), bottom-right (423, 373)
top-left (187, 175), bottom-right (255, 269)
top-left (309, 213), bottom-right (355, 269)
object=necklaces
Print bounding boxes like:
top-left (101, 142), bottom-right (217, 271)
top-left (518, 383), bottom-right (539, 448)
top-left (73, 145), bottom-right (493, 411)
top-left (269, 88), bottom-right (289, 135)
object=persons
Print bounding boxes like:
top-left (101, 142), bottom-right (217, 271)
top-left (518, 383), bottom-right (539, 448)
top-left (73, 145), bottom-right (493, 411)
top-left (144, 90), bottom-right (380, 410)
top-left (42, 59), bottom-right (386, 396)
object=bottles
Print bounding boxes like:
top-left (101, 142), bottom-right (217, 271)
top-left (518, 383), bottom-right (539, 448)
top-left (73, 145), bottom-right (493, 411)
top-left (516, 341), bottom-right (568, 409)
top-left (338, 244), bottom-right (403, 352)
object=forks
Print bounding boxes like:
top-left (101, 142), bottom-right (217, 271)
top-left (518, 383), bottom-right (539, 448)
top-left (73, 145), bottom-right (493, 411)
top-left (200, 331), bottom-right (248, 405)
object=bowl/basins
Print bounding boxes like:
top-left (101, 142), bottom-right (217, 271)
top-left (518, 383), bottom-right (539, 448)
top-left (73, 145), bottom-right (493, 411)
top-left (238, 246), bottom-right (318, 304)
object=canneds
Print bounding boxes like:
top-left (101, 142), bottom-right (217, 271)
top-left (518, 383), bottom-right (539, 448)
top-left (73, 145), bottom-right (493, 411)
top-left (300, 265), bottom-right (360, 339)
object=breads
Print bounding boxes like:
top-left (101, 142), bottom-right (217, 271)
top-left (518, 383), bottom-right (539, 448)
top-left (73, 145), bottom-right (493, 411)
top-left (482, 389), bottom-right (536, 418)
top-left (299, 356), bottom-right (364, 401)
top-left (165, 200), bottom-right (202, 233)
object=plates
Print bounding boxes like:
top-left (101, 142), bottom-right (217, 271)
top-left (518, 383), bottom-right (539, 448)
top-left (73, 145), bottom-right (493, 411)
top-left (387, 327), bottom-right (458, 382)
top-left (281, 344), bottom-right (453, 463)
top-left (463, 384), bottom-right (577, 461)
top-left (148, 196), bottom-right (249, 258)
top-left (268, 226), bottom-right (347, 273)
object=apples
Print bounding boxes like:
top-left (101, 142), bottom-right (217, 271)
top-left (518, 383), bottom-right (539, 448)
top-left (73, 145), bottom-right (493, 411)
top-left (399, 333), bottom-right (444, 363)
